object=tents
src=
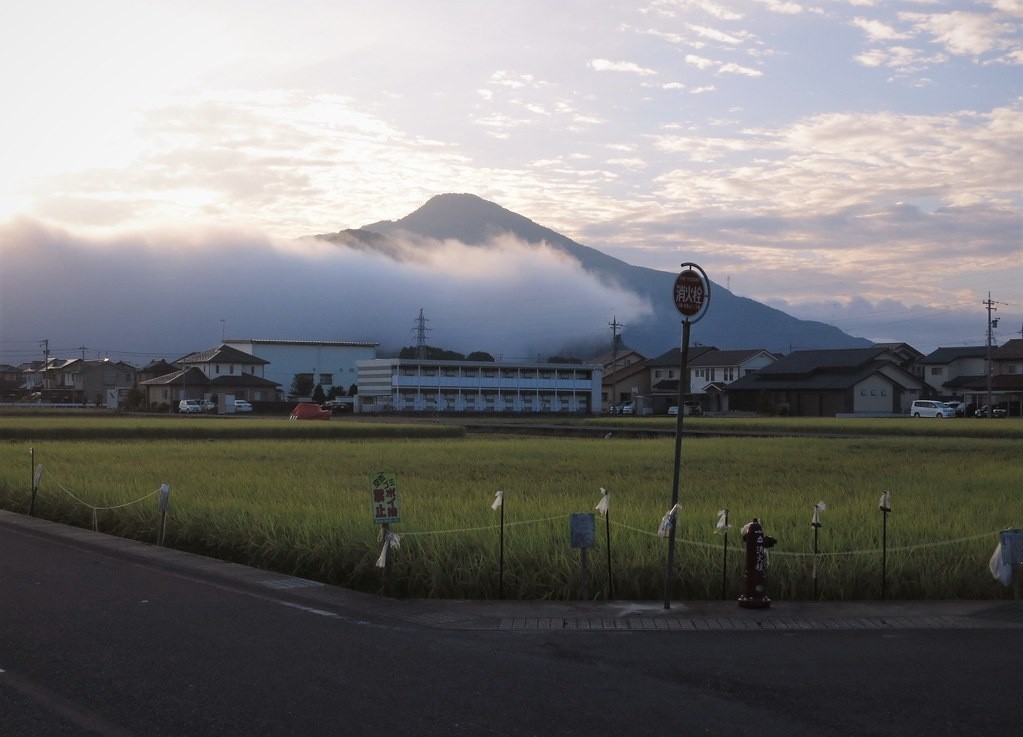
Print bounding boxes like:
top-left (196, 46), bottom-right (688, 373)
top-left (288, 403), bottom-right (332, 420)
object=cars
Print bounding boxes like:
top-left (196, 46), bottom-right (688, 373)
top-left (234, 399), bottom-right (253, 413)
top-left (992, 404), bottom-right (1008, 418)
top-left (178, 401), bottom-right (203, 414)
top-left (955, 401), bottom-right (974, 416)
top-left (943, 402), bottom-right (961, 412)
top-left (973, 404), bottom-right (992, 417)
top-left (320, 400), bottom-right (350, 412)
top-left (197, 399), bottom-right (217, 411)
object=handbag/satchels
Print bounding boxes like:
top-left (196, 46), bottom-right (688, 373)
top-left (989, 542), bottom-right (1013, 586)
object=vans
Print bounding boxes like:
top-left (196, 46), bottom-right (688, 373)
top-left (609, 400), bottom-right (627, 415)
top-left (668, 400), bottom-right (701, 414)
top-left (622, 401), bottom-right (636, 416)
top-left (910, 400), bottom-right (957, 419)
